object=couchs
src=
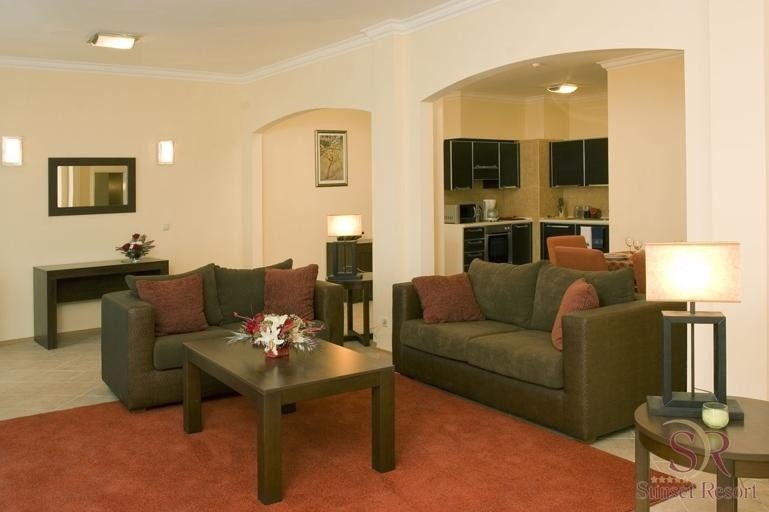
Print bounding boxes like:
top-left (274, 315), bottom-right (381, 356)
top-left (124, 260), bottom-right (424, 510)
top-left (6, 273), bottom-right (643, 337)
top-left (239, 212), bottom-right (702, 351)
top-left (391, 280), bottom-right (686, 442)
top-left (101, 278), bottom-right (345, 411)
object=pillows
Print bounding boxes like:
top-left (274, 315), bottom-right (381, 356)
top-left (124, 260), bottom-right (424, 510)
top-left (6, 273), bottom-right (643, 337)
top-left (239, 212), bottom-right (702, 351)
top-left (551, 279), bottom-right (597, 350)
top-left (531, 266), bottom-right (636, 331)
top-left (262, 263), bottom-right (321, 319)
top-left (135, 271), bottom-right (209, 335)
top-left (215, 257), bottom-right (294, 318)
top-left (414, 274), bottom-right (479, 323)
top-left (124, 263), bottom-right (217, 323)
top-left (467, 258), bottom-right (541, 327)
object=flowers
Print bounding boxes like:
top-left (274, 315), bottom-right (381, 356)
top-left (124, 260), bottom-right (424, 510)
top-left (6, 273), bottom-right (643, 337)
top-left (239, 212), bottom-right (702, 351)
top-left (115, 232), bottom-right (154, 259)
top-left (228, 313), bottom-right (323, 354)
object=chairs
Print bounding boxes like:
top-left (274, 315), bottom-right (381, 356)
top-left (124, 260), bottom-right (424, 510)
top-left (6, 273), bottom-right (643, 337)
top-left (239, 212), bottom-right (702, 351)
top-left (554, 245), bottom-right (607, 271)
top-left (547, 235), bottom-right (588, 265)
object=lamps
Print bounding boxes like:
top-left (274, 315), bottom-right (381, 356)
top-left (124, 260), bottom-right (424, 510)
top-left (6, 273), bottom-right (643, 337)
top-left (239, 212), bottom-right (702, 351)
top-left (86, 32), bottom-right (142, 51)
top-left (327, 215), bottom-right (364, 242)
top-left (548, 83), bottom-right (578, 95)
top-left (158, 140), bottom-right (177, 164)
top-left (1, 136), bottom-right (24, 164)
top-left (644, 242), bottom-right (746, 422)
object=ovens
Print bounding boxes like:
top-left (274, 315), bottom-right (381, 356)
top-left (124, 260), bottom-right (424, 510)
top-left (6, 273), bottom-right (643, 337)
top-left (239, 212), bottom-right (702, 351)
top-left (484, 233), bottom-right (513, 264)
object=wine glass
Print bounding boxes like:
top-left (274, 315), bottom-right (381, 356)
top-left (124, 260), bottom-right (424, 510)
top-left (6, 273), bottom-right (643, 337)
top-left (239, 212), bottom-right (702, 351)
top-left (624, 237), bottom-right (633, 254)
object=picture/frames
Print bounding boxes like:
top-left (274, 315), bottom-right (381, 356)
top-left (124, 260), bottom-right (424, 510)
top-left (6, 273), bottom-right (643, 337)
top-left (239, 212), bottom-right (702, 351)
top-left (315, 129), bottom-right (348, 188)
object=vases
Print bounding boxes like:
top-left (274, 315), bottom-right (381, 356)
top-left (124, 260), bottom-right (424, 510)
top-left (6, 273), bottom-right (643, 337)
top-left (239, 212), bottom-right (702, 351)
top-left (262, 344), bottom-right (291, 364)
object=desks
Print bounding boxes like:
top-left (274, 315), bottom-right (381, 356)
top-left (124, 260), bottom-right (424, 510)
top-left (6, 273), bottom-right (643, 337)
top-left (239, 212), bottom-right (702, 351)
top-left (31, 257), bottom-right (167, 350)
top-left (634, 395), bottom-right (769, 511)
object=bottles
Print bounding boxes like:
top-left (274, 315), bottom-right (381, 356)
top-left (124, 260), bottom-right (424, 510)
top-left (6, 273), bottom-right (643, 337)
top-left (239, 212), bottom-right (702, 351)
top-left (573, 205), bottom-right (590, 219)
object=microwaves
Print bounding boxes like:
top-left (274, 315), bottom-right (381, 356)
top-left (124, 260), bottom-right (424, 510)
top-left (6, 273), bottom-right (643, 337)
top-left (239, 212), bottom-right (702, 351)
top-left (444, 202), bottom-right (479, 224)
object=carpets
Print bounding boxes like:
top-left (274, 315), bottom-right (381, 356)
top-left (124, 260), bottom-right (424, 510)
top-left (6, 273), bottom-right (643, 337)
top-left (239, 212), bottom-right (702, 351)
top-left (1, 374), bottom-right (696, 512)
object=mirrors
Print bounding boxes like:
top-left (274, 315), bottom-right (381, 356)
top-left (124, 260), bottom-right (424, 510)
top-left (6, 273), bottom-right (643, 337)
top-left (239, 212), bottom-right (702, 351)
top-left (47, 157), bottom-right (136, 217)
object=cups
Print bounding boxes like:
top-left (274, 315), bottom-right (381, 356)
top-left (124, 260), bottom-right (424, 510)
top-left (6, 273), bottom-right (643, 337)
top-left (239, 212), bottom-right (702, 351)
top-left (633, 240), bottom-right (643, 250)
top-left (702, 402), bottom-right (730, 430)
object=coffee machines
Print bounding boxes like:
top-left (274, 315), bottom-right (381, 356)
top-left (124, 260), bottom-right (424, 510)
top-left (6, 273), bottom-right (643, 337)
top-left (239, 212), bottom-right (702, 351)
top-left (482, 199), bottom-right (499, 222)
